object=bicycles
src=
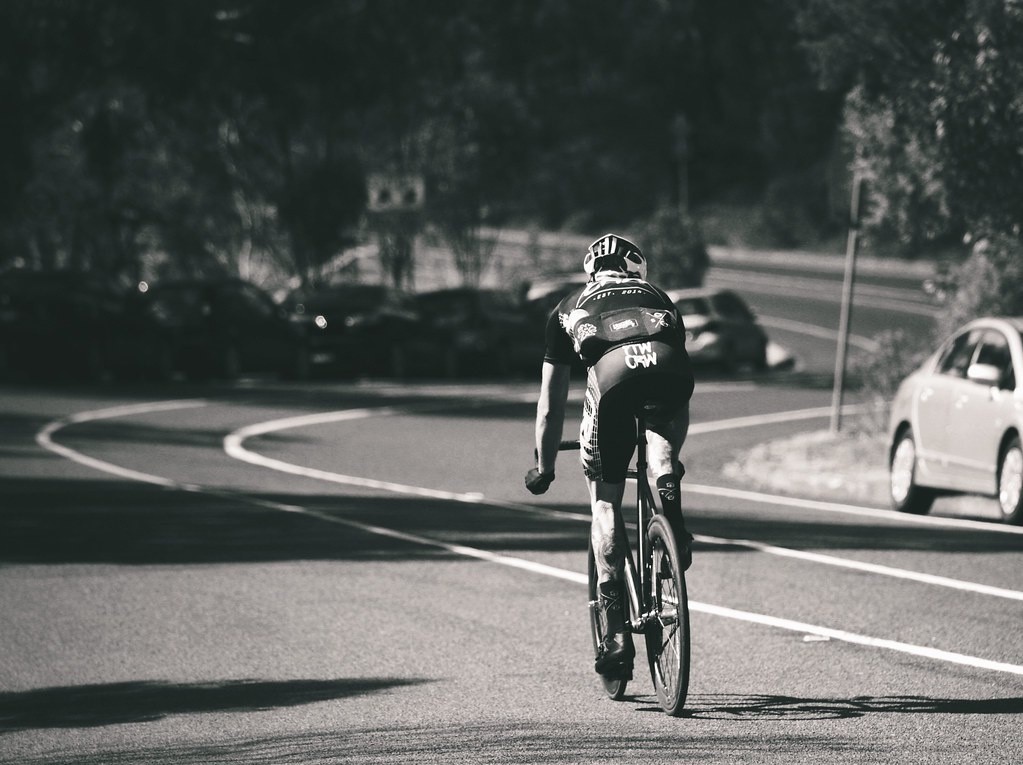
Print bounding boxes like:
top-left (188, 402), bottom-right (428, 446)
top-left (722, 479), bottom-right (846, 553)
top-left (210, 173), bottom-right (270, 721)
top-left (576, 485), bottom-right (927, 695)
top-left (534, 399), bottom-right (692, 716)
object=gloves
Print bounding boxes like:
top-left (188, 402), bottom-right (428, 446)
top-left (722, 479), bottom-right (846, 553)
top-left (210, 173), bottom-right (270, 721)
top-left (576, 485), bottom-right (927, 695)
top-left (525, 469), bottom-right (553, 496)
top-left (678, 460), bottom-right (685, 479)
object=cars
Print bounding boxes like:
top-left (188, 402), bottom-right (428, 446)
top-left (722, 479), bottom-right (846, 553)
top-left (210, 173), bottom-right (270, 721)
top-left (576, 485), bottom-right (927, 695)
top-left (884, 317), bottom-right (1023, 526)
top-left (666, 286), bottom-right (771, 378)
top-left (281, 283), bottom-right (411, 383)
top-left (0, 269), bottom-right (281, 394)
top-left (406, 273), bottom-right (587, 382)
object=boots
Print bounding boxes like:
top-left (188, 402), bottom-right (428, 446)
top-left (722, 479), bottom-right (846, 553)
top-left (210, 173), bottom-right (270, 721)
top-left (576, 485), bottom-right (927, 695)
top-left (657, 474), bottom-right (694, 576)
top-left (594, 578), bottom-right (636, 675)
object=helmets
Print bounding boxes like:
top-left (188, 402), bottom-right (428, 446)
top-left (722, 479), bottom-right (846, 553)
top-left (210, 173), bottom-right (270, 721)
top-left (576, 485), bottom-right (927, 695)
top-left (584, 234), bottom-right (646, 281)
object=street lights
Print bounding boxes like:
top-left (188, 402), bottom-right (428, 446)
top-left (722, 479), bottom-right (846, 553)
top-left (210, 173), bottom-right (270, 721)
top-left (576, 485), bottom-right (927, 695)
top-left (831, 86), bottom-right (870, 433)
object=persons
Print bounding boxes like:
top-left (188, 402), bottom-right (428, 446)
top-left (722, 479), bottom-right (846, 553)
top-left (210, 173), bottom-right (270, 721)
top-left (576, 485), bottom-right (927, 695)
top-left (523, 233), bottom-right (697, 676)
